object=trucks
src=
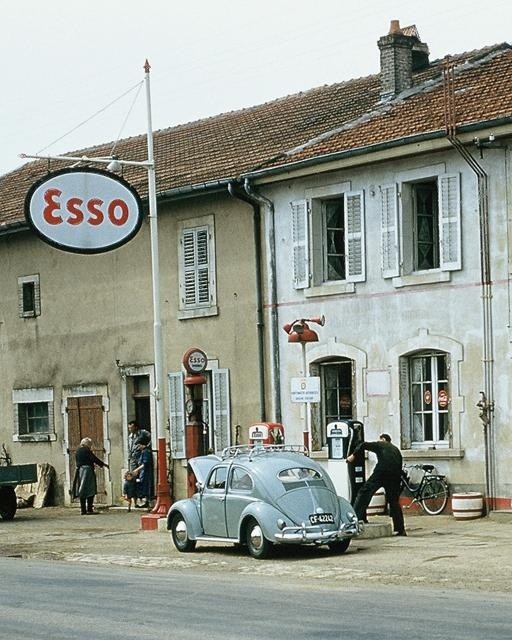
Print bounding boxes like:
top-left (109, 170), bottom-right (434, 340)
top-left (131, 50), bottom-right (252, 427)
top-left (0, 443), bottom-right (39, 520)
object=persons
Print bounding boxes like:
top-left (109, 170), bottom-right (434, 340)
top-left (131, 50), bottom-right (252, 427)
top-left (70, 436), bottom-right (110, 516)
top-left (132, 436), bottom-right (153, 509)
top-left (128, 421), bottom-right (151, 474)
top-left (123, 471), bottom-right (138, 515)
top-left (347, 433), bottom-right (407, 539)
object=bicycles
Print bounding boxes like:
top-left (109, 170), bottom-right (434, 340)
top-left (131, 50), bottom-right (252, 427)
top-left (385, 463), bottom-right (452, 515)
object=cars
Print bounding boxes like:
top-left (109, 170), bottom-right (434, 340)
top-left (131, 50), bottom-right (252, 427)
top-left (166, 443), bottom-right (365, 559)
